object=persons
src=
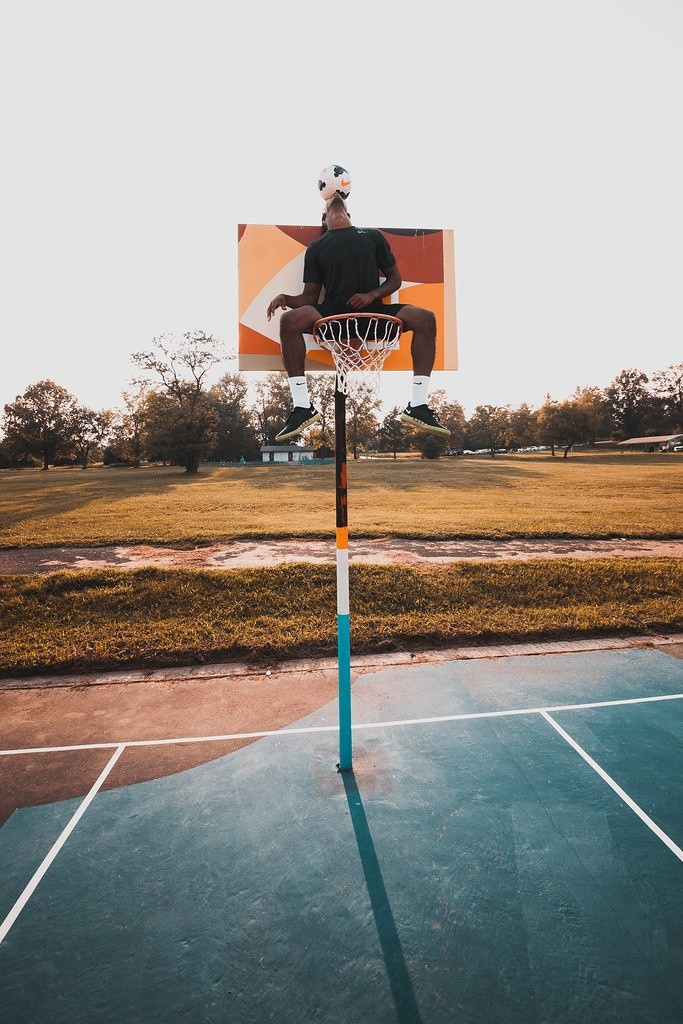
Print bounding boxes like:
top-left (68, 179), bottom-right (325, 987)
top-left (266, 192), bottom-right (451, 442)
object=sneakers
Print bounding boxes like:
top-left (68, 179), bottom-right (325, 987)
top-left (275, 401), bottom-right (321, 442)
top-left (401, 401), bottom-right (451, 437)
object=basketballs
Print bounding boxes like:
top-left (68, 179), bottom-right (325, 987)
top-left (317, 164), bottom-right (353, 202)
